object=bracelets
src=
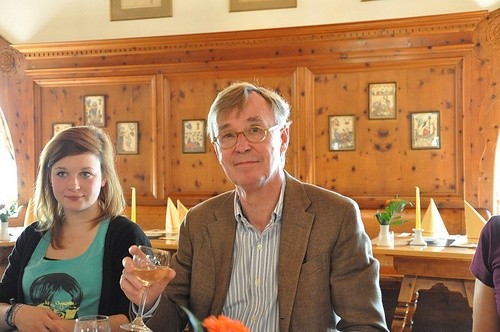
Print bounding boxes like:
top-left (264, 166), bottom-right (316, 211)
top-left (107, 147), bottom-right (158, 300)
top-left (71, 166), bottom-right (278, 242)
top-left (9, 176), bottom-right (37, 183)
top-left (5, 303), bottom-right (25, 327)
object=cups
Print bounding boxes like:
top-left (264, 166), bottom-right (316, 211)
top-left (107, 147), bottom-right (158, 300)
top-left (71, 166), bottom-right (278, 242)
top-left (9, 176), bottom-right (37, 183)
top-left (387, 231), bottom-right (395, 249)
top-left (73, 314), bottom-right (112, 332)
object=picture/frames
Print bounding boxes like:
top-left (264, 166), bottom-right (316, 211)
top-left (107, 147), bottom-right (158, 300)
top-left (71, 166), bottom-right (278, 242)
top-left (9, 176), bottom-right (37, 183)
top-left (182, 119), bottom-right (206, 153)
top-left (328, 115), bottom-right (356, 150)
top-left (410, 110), bottom-right (440, 150)
top-left (115, 122), bottom-right (139, 155)
top-left (111, 0), bottom-right (173, 21)
top-left (368, 82), bottom-right (397, 120)
top-left (82, 95), bottom-right (105, 127)
top-left (52, 123), bottom-right (73, 138)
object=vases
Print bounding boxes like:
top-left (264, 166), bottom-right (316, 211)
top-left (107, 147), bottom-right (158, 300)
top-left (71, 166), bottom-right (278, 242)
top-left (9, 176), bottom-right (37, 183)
top-left (378, 225), bottom-right (392, 247)
top-left (0, 221), bottom-right (10, 241)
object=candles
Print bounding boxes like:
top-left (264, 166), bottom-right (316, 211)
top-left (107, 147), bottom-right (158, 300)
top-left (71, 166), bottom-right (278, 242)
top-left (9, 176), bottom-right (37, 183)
top-left (416, 186), bottom-right (421, 229)
top-left (131, 187), bottom-right (136, 223)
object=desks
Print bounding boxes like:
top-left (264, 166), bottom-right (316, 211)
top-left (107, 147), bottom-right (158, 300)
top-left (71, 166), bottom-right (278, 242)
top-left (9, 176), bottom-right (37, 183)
top-left (370, 233), bottom-right (477, 332)
top-left (141, 229), bottom-right (179, 250)
top-left (0, 226), bottom-right (26, 274)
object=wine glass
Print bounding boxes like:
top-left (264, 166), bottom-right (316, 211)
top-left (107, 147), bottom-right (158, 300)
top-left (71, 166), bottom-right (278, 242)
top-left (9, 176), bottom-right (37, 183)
top-left (119, 245), bottom-right (171, 332)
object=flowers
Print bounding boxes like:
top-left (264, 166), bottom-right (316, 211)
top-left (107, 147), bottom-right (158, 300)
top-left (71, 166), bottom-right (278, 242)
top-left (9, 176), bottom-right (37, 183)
top-left (201, 314), bottom-right (250, 332)
top-left (0, 197), bottom-right (24, 222)
top-left (374, 194), bottom-right (413, 225)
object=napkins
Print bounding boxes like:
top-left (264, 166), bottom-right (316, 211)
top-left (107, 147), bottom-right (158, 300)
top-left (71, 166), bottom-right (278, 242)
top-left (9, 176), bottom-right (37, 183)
top-left (421, 198), bottom-right (449, 235)
top-left (166, 196), bottom-right (188, 231)
top-left (463, 201), bottom-right (486, 242)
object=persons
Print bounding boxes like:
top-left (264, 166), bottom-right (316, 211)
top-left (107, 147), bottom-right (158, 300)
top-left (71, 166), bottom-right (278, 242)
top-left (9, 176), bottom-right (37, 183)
top-left (120, 74), bottom-right (389, 332)
top-left (469, 215), bottom-right (500, 332)
top-left (0, 124), bottom-right (154, 332)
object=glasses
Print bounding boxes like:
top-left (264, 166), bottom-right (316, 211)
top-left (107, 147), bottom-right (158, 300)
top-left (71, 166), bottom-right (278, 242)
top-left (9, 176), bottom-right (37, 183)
top-left (214, 124), bottom-right (285, 149)
